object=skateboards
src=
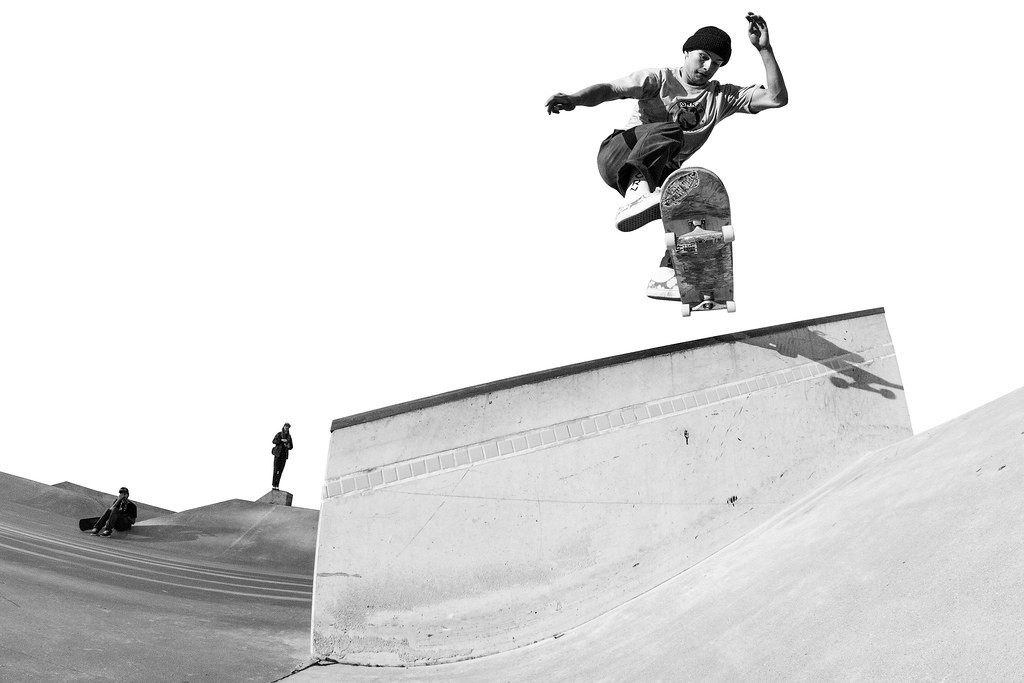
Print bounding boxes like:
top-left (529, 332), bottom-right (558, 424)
top-left (659, 165), bottom-right (737, 319)
top-left (79, 516), bottom-right (101, 531)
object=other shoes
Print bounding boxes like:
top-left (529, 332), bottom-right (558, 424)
top-left (272, 486), bottom-right (279, 490)
top-left (100, 530), bottom-right (111, 537)
top-left (91, 528), bottom-right (100, 536)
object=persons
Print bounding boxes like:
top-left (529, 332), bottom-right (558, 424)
top-left (272, 422), bottom-right (293, 490)
top-left (546, 12), bottom-right (789, 302)
top-left (90, 486), bottom-right (137, 537)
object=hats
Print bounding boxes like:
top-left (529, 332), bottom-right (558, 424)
top-left (285, 423), bottom-right (291, 427)
top-left (682, 26), bottom-right (732, 67)
top-left (119, 487), bottom-right (130, 497)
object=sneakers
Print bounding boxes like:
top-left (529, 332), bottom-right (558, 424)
top-left (614, 186), bottom-right (662, 232)
top-left (647, 276), bottom-right (681, 299)
